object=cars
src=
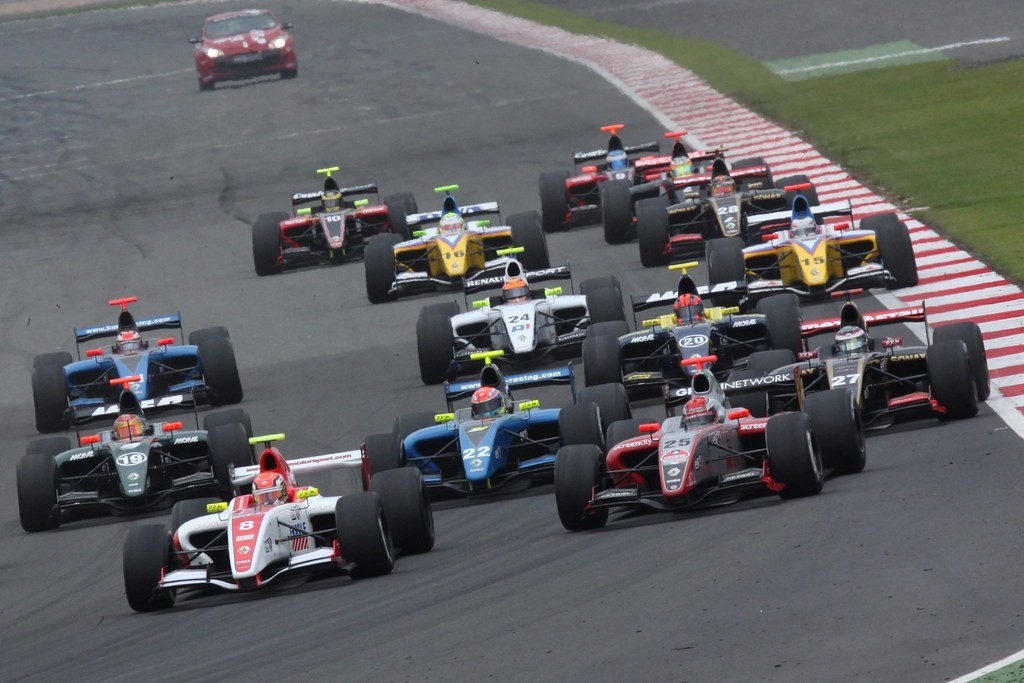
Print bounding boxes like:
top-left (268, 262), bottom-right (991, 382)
top-left (191, 9), bottom-right (298, 91)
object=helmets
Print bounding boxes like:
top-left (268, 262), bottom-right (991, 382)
top-left (503, 278), bottom-right (530, 302)
top-left (470, 387), bottom-right (507, 419)
top-left (682, 397), bottom-right (726, 429)
top-left (116, 330), bottom-right (141, 356)
top-left (835, 326), bottom-right (869, 354)
top-left (669, 156), bottom-right (695, 177)
top-left (606, 150), bottom-right (629, 171)
top-left (673, 294), bottom-right (707, 326)
top-left (710, 175), bottom-right (737, 197)
top-left (791, 216), bottom-right (817, 238)
top-left (438, 212), bottom-right (465, 234)
top-left (321, 189), bottom-right (344, 212)
top-left (113, 414), bottom-right (146, 444)
top-left (252, 473), bottom-right (289, 505)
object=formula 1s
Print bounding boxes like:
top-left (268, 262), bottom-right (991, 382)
top-left (364, 185), bottom-right (552, 304)
top-left (744, 286), bottom-right (991, 430)
top-left (251, 164), bottom-right (423, 275)
top-left (540, 122), bottom-right (825, 267)
top-left (17, 376), bottom-right (255, 532)
top-left (33, 294), bottom-right (245, 435)
top-left (706, 183), bottom-right (918, 308)
top-left (416, 247), bottom-right (627, 386)
top-left (553, 356), bottom-right (866, 531)
top-left (393, 350), bottom-right (628, 505)
top-left (583, 260), bottom-right (804, 400)
top-left (123, 429), bottom-right (434, 612)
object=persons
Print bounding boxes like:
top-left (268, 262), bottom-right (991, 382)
top-left (670, 156), bottom-right (694, 178)
top-left (438, 212), bottom-right (466, 234)
top-left (711, 175), bottom-right (735, 195)
top-left (835, 325), bottom-right (869, 355)
top-left (251, 472), bottom-right (288, 504)
top-left (471, 386), bottom-right (505, 420)
top-left (673, 293), bottom-right (705, 325)
top-left (117, 330), bottom-right (142, 354)
top-left (502, 278), bottom-right (528, 303)
top-left (113, 414), bottom-right (146, 441)
top-left (790, 217), bottom-right (821, 238)
top-left (322, 190), bottom-right (343, 212)
top-left (605, 151), bottom-right (629, 171)
top-left (682, 397), bottom-right (718, 429)
